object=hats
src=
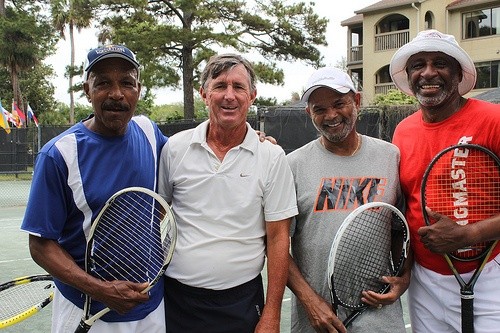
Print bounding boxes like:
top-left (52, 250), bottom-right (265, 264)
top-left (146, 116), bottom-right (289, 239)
top-left (302, 66), bottom-right (357, 109)
top-left (83, 45), bottom-right (140, 83)
top-left (389, 29), bottom-right (477, 97)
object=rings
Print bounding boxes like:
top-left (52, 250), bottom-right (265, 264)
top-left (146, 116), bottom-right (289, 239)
top-left (377, 304), bottom-right (382, 309)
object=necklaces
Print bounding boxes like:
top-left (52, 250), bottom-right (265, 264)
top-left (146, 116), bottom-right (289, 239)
top-left (352, 135), bottom-right (359, 155)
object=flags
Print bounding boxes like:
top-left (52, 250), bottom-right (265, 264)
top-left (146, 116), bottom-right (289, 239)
top-left (27, 105), bottom-right (38, 126)
top-left (0, 102), bottom-right (26, 134)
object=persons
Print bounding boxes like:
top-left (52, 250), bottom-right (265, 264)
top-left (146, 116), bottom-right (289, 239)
top-left (265, 66), bottom-right (411, 333)
top-left (389, 30), bottom-right (500, 333)
top-left (20, 44), bottom-right (277, 333)
top-left (158, 53), bottom-right (299, 333)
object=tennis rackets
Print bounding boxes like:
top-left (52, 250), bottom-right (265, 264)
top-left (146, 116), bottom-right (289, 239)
top-left (420, 143), bottom-right (500, 333)
top-left (74, 186), bottom-right (178, 333)
top-left (327, 201), bottom-right (411, 328)
top-left (0, 274), bottom-right (54, 330)
